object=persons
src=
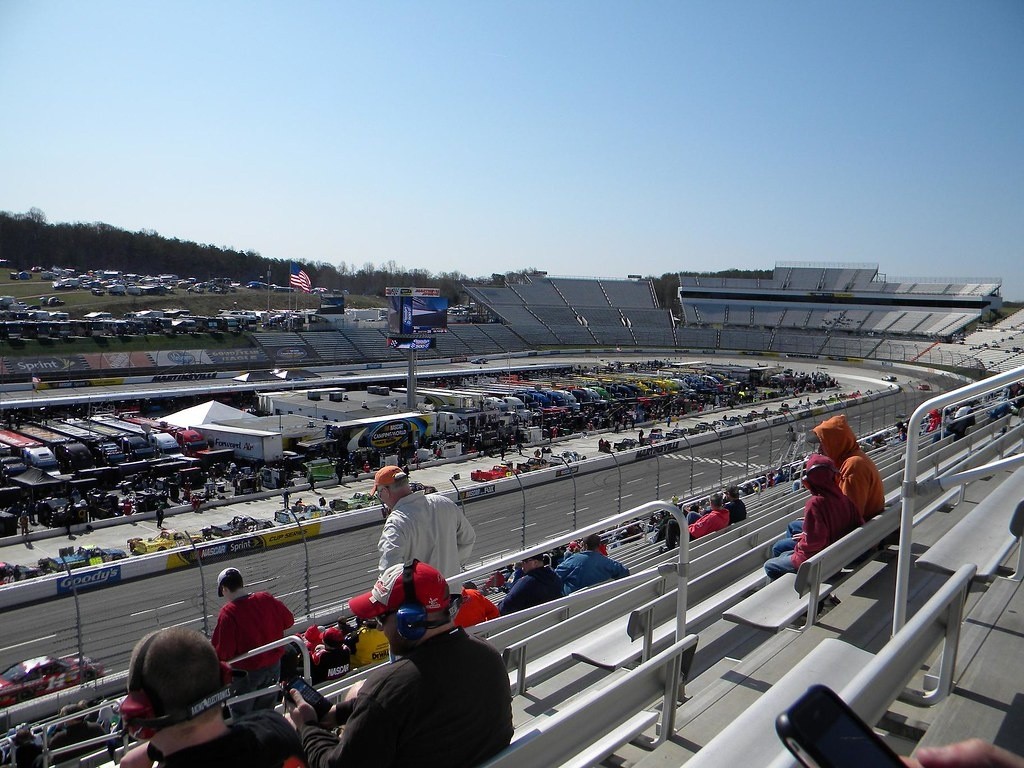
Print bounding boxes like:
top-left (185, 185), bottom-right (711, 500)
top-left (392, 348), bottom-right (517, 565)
top-left (112, 627), bottom-right (308, 768)
top-left (0, 336), bottom-right (1024, 768)
top-left (370, 465), bottom-right (476, 595)
top-left (280, 562), bottom-right (514, 768)
top-left (904, 737), bottom-right (1024, 768)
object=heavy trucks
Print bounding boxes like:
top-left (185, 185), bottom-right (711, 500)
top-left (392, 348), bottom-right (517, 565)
top-left (393, 366), bottom-right (740, 426)
top-left (0, 410), bottom-right (212, 486)
top-left (297, 410), bottom-right (469, 459)
top-left (750, 365), bottom-right (784, 383)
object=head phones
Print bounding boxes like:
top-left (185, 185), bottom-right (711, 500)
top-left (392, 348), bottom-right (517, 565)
top-left (397, 558), bottom-right (427, 640)
top-left (120, 661), bottom-right (249, 740)
top-left (802, 463), bottom-right (841, 494)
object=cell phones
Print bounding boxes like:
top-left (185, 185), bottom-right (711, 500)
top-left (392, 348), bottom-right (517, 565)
top-left (283, 676), bottom-right (332, 720)
top-left (775, 683), bottom-right (909, 768)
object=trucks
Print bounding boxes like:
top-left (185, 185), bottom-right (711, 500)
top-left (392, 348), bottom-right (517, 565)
top-left (346, 309), bottom-right (389, 323)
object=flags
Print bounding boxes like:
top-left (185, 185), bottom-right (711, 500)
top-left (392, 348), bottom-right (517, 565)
top-left (290, 261), bottom-right (312, 293)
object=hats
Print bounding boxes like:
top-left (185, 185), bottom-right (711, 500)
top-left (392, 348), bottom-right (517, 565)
top-left (325, 627), bottom-right (346, 648)
top-left (370, 466), bottom-right (407, 496)
top-left (98, 707), bottom-right (115, 721)
top-left (523, 553), bottom-right (543, 560)
top-left (217, 568), bottom-right (242, 597)
top-left (806, 431), bottom-right (820, 442)
top-left (348, 562), bottom-right (451, 618)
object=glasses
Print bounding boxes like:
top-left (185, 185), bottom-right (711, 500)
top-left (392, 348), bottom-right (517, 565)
top-left (375, 607), bottom-right (396, 627)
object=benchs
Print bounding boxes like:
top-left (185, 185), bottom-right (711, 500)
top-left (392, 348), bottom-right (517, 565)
top-left (0, 438), bottom-right (1024, 768)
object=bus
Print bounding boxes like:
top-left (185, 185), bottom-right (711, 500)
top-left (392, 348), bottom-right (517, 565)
top-left (0, 308), bottom-right (315, 339)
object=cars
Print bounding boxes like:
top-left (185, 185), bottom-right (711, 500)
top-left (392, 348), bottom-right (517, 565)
top-left (917, 383), bottom-right (930, 390)
top-left (0, 293), bottom-right (66, 309)
top-left (10, 267), bottom-right (324, 296)
top-left (471, 451), bottom-right (584, 480)
top-left (39, 478), bottom-right (438, 574)
top-left (447, 305), bottom-right (476, 315)
top-left (1, 650), bottom-right (103, 709)
top-left (0, 561), bottom-right (44, 585)
top-left (882, 374), bottom-right (897, 381)
